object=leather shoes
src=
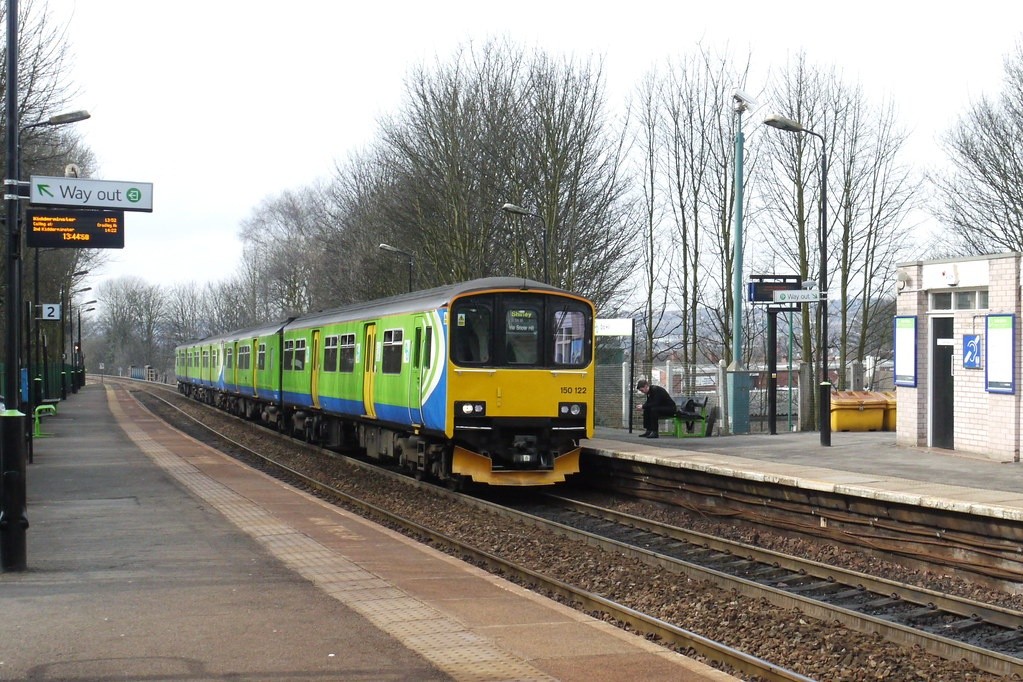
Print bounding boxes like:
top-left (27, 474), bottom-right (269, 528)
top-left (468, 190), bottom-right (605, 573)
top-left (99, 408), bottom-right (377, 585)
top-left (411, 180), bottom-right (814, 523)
top-left (639, 428), bottom-right (659, 438)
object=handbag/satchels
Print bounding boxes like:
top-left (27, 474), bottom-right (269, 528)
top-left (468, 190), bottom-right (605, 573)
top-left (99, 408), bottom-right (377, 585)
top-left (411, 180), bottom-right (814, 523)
top-left (681, 399), bottom-right (695, 430)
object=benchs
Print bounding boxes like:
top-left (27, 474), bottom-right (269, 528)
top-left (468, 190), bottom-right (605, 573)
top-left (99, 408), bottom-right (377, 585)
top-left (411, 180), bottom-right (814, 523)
top-left (654, 404), bottom-right (707, 438)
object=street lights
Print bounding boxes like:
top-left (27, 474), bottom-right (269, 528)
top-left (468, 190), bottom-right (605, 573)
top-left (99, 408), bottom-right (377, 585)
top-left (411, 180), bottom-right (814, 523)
top-left (17, 109), bottom-right (91, 356)
top-left (378, 244), bottom-right (412, 292)
top-left (764, 115), bottom-right (834, 446)
top-left (502, 202), bottom-right (549, 284)
top-left (59, 268), bottom-right (96, 400)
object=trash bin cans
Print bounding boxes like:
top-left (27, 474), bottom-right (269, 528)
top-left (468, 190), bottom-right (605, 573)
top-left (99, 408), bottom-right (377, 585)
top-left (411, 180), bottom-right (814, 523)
top-left (830, 389), bottom-right (887, 432)
top-left (881, 391), bottom-right (896, 432)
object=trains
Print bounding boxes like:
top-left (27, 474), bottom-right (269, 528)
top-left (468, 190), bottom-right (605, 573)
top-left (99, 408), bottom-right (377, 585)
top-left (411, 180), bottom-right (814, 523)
top-left (173, 278), bottom-right (597, 506)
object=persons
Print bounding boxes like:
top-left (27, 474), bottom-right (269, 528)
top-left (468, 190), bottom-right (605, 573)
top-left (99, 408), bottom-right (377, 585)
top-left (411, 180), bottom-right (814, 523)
top-left (637, 379), bottom-right (678, 439)
